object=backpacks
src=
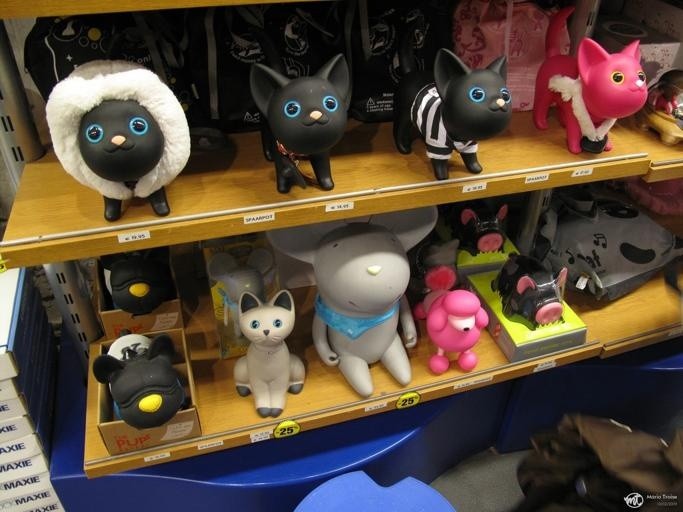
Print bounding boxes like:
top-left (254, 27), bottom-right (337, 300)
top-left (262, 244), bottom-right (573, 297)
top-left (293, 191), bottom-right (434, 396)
top-left (516, 415), bottom-right (682, 512)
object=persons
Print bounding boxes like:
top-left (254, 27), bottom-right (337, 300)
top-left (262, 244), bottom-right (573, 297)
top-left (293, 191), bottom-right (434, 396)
top-left (45, 36), bottom-right (683, 429)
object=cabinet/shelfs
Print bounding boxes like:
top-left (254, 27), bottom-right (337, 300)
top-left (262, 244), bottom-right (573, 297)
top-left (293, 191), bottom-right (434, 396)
top-left (0, 0), bottom-right (648, 480)
top-left (528, 0), bottom-right (683, 402)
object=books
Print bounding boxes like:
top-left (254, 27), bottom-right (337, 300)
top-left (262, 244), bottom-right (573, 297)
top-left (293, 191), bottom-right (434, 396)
top-left (0, 268), bottom-right (67, 512)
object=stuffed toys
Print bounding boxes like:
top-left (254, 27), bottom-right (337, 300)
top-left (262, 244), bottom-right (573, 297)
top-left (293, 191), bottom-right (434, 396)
top-left (45, 36), bottom-right (683, 429)
